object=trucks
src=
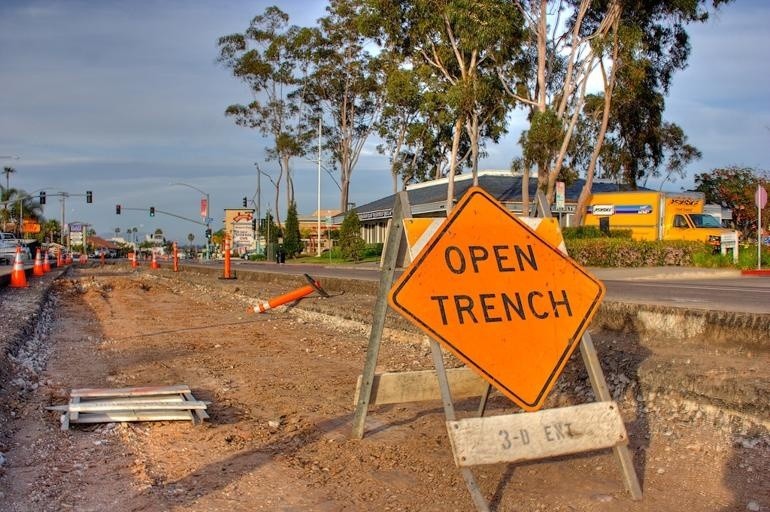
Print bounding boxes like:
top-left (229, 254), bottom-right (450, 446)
top-left (582, 189), bottom-right (742, 246)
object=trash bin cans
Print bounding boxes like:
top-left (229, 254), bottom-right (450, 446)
top-left (276, 251), bottom-right (285, 263)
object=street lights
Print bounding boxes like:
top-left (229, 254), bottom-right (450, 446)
top-left (243, 196), bottom-right (247, 207)
top-left (168, 182), bottom-right (210, 260)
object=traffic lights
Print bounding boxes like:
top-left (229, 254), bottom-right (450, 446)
top-left (150, 207), bottom-right (154, 217)
top-left (116, 205), bottom-right (121, 214)
top-left (205, 229), bottom-right (212, 239)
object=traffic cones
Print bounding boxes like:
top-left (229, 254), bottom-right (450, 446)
top-left (7, 246), bottom-right (160, 290)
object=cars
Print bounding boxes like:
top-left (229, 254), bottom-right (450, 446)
top-left (0, 232), bottom-right (117, 266)
top-left (137, 248), bottom-right (186, 260)
top-left (240, 250), bottom-right (264, 260)
